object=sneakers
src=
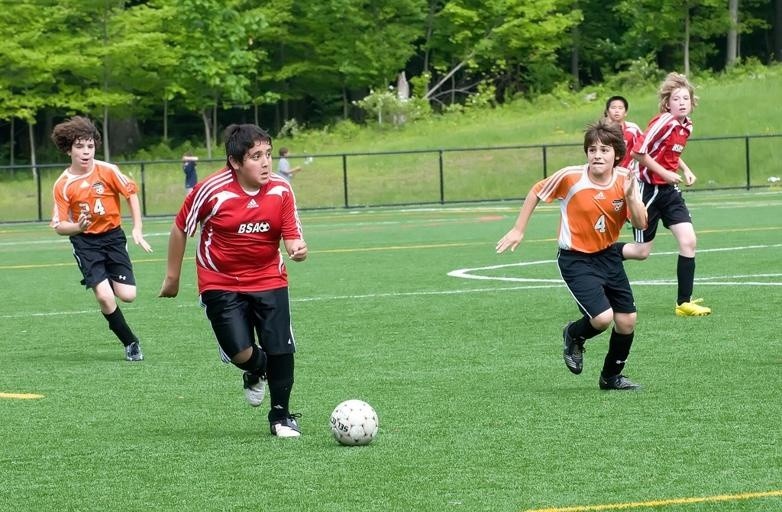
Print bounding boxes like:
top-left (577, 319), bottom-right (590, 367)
top-left (675, 298), bottom-right (711, 316)
top-left (599, 374), bottom-right (639, 389)
top-left (244, 371), bottom-right (266, 406)
top-left (266, 412), bottom-right (302, 437)
top-left (563, 321), bottom-right (585, 374)
top-left (126, 341), bottom-right (144, 360)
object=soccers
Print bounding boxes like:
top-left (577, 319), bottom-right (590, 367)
top-left (331, 401), bottom-right (378, 446)
top-left (260, 221), bottom-right (270, 233)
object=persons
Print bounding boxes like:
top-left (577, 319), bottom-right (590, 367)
top-left (605, 96), bottom-right (644, 229)
top-left (616, 72), bottom-right (711, 316)
top-left (182, 152), bottom-right (198, 193)
top-left (159, 124), bottom-right (309, 437)
top-left (496, 121), bottom-right (648, 390)
top-left (278, 147), bottom-right (301, 183)
top-left (49, 117), bottom-right (154, 361)
